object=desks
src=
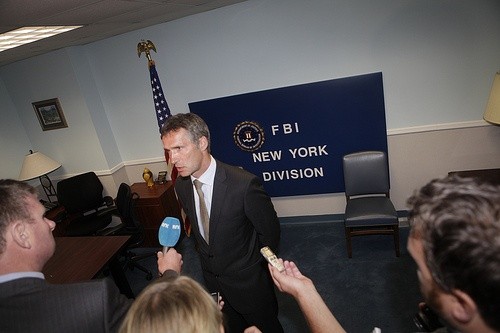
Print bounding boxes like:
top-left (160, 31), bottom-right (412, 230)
top-left (114, 182), bottom-right (184, 235)
top-left (41, 236), bottom-right (139, 299)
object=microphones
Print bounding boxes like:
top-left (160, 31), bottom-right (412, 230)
top-left (159, 217), bottom-right (181, 255)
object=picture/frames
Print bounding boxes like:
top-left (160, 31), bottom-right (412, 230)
top-left (32, 97), bottom-right (69, 132)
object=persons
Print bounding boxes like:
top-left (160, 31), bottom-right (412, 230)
top-left (0, 177), bottom-right (500, 333)
top-left (160, 114), bottom-right (286, 333)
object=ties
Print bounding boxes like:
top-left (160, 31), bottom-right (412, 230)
top-left (193, 178), bottom-right (209, 245)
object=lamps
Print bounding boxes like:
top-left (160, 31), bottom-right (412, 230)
top-left (18, 149), bottom-right (63, 203)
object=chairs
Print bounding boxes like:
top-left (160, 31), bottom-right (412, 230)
top-left (343, 151), bottom-right (401, 260)
top-left (56, 172), bottom-right (156, 282)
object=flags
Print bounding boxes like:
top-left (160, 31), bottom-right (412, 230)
top-left (149, 65), bottom-right (192, 239)
